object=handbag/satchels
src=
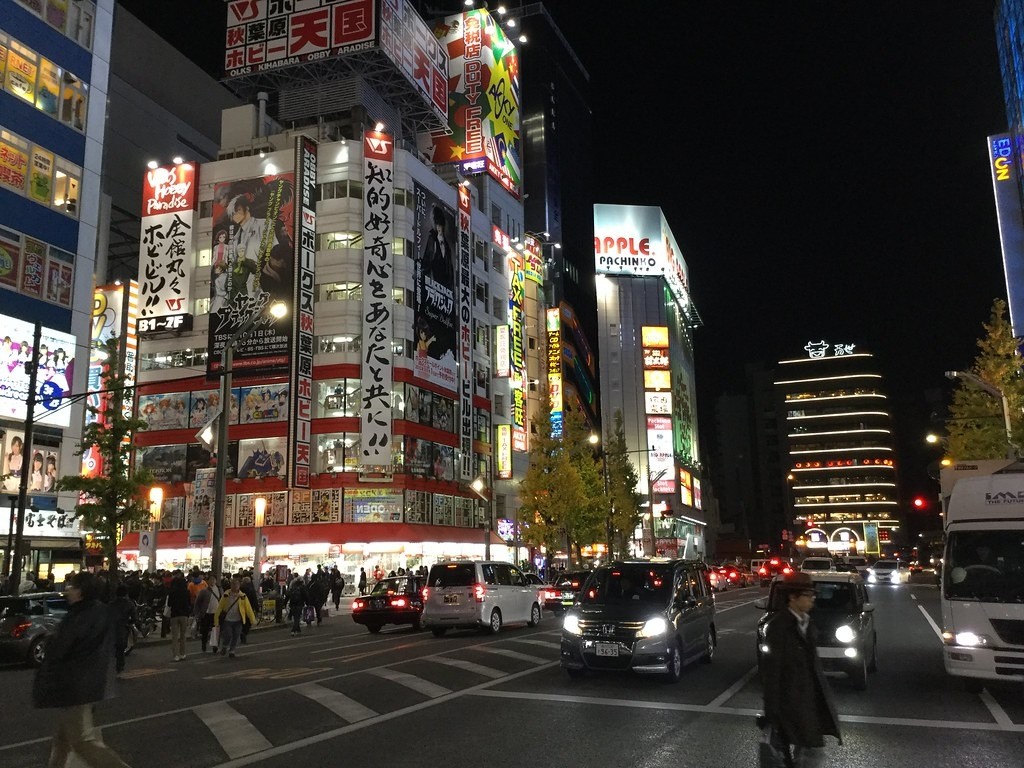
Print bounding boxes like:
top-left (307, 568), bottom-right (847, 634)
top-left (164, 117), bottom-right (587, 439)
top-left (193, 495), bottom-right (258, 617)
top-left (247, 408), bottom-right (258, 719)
top-left (218, 610), bottom-right (227, 621)
top-left (210, 626), bottom-right (219, 646)
top-left (303, 607), bottom-right (314, 621)
top-left (162, 595), bottom-right (171, 619)
top-left (320, 606), bottom-right (331, 617)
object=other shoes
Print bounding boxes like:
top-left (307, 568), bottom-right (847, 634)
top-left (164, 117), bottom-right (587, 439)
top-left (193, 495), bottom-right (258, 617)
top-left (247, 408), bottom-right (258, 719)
top-left (315, 622), bottom-right (320, 626)
top-left (213, 647), bottom-right (218, 653)
top-left (203, 648), bottom-right (206, 651)
top-left (296, 632), bottom-right (300, 637)
top-left (220, 649), bottom-right (226, 656)
top-left (229, 653), bottom-right (235, 658)
top-left (180, 655), bottom-right (187, 659)
top-left (174, 655), bottom-right (179, 662)
top-left (117, 672), bottom-right (130, 679)
top-left (335, 606), bottom-right (338, 610)
top-left (291, 632), bottom-right (295, 637)
top-left (240, 632), bottom-right (246, 644)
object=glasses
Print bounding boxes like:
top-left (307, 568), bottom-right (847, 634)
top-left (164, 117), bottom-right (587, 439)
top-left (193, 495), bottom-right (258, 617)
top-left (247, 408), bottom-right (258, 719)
top-left (801, 592), bottom-right (817, 599)
top-left (65, 585), bottom-right (73, 591)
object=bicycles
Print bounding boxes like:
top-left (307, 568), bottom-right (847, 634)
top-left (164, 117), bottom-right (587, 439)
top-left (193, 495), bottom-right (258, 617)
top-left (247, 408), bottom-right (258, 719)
top-left (131, 600), bottom-right (154, 639)
top-left (254, 600), bottom-right (275, 625)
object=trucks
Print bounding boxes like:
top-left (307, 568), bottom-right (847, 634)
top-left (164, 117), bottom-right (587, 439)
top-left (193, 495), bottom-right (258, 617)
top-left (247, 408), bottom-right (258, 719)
top-left (938, 459), bottom-right (1024, 696)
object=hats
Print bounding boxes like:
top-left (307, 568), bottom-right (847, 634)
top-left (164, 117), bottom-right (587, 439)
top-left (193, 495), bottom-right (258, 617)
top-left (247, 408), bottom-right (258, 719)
top-left (293, 573), bottom-right (299, 576)
top-left (375, 565), bottom-right (379, 568)
top-left (775, 572), bottom-right (821, 593)
top-left (26, 571), bottom-right (34, 581)
top-left (324, 566), bottom-right (329, 569)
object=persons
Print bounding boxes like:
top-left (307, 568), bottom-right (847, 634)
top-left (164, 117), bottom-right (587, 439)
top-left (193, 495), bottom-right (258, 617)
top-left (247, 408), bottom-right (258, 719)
top-left (194, 488), bottom-right (210, 520)
top-left (3, 436), bottom-right (23, 492)
top-left (0, 336), bottom-right (69, 392)
top-left (44, 455), bottom-right (56, 492)
top-left (33, 574), bottom-right (130, 768)
top-left (30, 453), bottom-right (44, 492)
top-left (141, 535), bottom-right (150, 555)
top-left (522, 558), bottom-right (565, 579)
top-left (762, 572), bottom-right (844, 768)
top-left (373, 565), bottom-right (429, 582)
top-left (358, 567), bottom-right (367, 595)
top-left (0, 564), bottom-right (345, 677)
top-left (263, 538), bottom-right (267, 556)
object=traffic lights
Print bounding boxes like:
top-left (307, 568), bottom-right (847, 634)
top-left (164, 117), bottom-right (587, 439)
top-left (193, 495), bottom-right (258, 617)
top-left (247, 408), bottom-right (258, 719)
top-left (914, 498), bottom-right (923, 507)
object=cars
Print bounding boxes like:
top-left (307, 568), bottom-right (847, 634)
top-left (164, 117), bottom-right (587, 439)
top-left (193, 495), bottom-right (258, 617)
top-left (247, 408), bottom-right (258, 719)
top-left (755, 572), bottom-right (879, 692)
top-left (352, 575), bottom-right (430, 634)
top-left (512, 573), bottom-right (553, 609)
top-left (866, 561), bottom-right (912, 586)
top-left (544, 570), bottom-right (593, 616)
top-left (705, 562), bottom-right (756, 591)
top-left (760, 560), bottom-right (793, 588)
top-left (0, 592), bottom-right (138, 668)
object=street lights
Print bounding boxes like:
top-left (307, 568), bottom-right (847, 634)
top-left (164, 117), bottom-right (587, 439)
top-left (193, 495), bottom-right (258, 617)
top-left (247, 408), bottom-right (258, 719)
top-left (148, 487), bottom-right (163, 573)
top-left (945, 370), bottom-right (1013, 445)
top-left (210, 299), bottom-right (287, 586)
top-left (253, 497), bottom-right (267, 591)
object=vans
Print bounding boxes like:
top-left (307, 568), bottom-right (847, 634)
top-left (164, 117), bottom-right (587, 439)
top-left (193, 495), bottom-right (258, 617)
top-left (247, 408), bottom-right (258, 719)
top-left (797, 556), bottom-right (838, 572)
top-left (560, 558), bottom-right (718, 684)
top-left (838, 554), bottom-right (872, 575)
top-left (421, 560), bottom-right (542, 637)
top-left (750, 559), bottom-right (764, 573)
